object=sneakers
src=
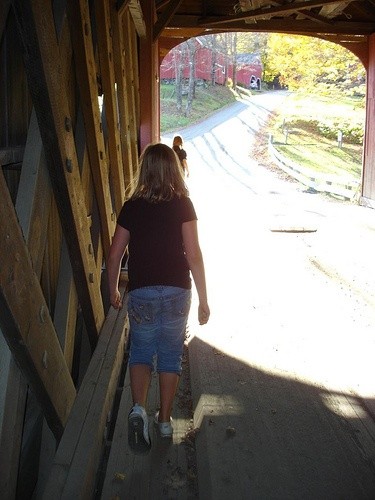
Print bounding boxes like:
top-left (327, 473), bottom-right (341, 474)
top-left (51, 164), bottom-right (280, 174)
top-left (127, 405), bottom-right (151, 453)
top-left (154, 410), bottom-right (173, 439)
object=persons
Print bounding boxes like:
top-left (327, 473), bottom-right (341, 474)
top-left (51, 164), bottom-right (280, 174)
top-left (104, 144), bottom-right (210, 453)
top-left (170, 136), bottom-right (190, 178)
